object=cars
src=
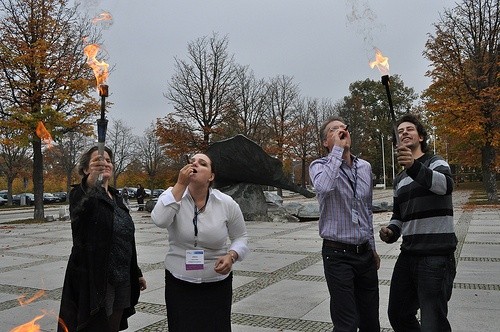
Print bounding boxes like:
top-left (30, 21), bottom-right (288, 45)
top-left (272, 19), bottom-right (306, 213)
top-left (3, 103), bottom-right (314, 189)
top-left (296, 183), bottom-right (316, 194)
top-left (0, 187), bottom-right (68, 206)
top-left (117, 188), bottom-right (165, 199)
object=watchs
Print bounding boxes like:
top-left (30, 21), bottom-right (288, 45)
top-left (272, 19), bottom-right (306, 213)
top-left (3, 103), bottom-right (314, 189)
top-left (228, 252), bottom-right (236, 264)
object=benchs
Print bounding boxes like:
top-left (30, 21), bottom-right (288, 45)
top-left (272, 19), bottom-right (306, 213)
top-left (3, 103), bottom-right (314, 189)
top-left (127, 203), bottom-right (146, 212)
top-left (374, 184), bottom-right (385, 188)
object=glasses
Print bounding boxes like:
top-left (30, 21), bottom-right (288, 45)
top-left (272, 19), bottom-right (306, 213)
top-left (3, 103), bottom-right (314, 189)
top-left (325, 124), bottom-right (348, 139)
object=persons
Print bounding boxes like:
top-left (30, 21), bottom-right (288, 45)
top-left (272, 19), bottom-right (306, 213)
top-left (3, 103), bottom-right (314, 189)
top-left (135, 183), bottom-right (146, 211)
top-left (149, 152), bottom-right (251, 331)
top-left (309, 116), bottom-right (380, 332)
top-left (58, 145), bottom-right (147, 332)
top-left (379, 115), bottom-right (459, 332)
top-left (120, 187), bottom-right (132, 212)
